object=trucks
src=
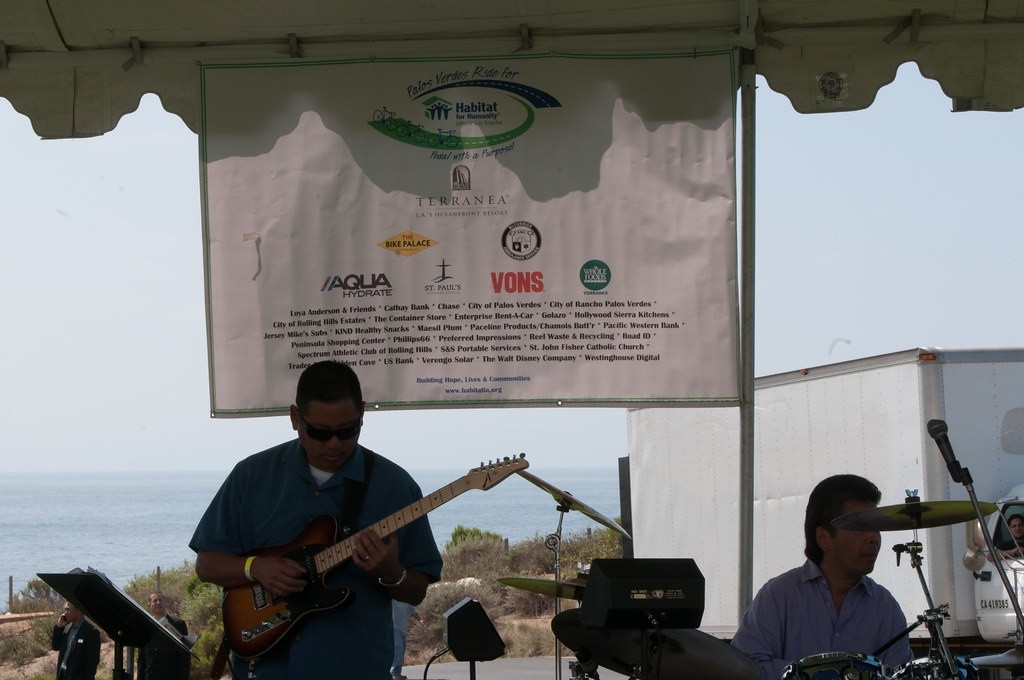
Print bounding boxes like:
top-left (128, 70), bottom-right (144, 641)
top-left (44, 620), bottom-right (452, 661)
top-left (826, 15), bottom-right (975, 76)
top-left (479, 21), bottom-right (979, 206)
top-left (624, 346), bottom-right (1024, 680)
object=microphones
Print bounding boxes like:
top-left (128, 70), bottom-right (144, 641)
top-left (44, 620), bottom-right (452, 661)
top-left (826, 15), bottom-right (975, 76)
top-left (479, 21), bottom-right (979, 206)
top-left (927, 417), bottom-right (961, 484)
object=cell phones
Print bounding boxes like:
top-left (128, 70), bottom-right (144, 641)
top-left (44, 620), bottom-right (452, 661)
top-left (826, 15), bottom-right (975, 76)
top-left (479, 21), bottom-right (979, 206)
top-left (63, 616), bottom-right (67, 622)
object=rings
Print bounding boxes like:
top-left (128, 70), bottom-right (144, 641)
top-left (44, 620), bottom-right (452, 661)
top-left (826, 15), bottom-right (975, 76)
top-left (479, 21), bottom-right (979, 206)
top-left (363, 555), bottom-right (369, 560)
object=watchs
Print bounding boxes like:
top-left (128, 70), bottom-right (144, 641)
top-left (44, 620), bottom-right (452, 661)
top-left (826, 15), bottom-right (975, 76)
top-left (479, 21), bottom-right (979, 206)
top-left (379, 564), bottom-right (408, 588)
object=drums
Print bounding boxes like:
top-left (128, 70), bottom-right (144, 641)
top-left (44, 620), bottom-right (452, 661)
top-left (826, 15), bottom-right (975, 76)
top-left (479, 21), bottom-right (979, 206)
top-left (780, 648), bottom-right (958, 680)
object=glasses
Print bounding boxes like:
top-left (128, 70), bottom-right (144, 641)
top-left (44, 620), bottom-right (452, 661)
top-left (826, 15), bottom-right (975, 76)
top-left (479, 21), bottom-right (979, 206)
top-left (298, 409), bottom-right (361, 440)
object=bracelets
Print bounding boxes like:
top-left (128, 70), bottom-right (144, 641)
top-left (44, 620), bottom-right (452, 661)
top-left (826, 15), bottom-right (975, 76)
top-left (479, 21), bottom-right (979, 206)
top-left (244, 556), bottom-right (257, 582)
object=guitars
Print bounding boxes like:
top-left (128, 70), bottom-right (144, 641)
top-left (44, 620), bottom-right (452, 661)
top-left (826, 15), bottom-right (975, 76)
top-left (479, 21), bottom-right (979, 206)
top-left (221, 453), bottom-right (530, 657)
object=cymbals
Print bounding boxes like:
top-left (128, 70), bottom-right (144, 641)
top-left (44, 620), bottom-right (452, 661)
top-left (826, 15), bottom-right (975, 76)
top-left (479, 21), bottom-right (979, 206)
top-left (515, 467), bottom-right (631, 540)
top-left (831, 502), bottom-right (998, 531)
top-left (965, 648), bottom-right (1024, 670)
top-left (497, 577), bottom-right (585, 601)
top-left (551, 608), bottom-right (765, 680)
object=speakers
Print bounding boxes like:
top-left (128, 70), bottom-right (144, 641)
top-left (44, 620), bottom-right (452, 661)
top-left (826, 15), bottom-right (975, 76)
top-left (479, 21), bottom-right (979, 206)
top-left (441, 597), bottom-right (506, 661)
top-left (578, 557), bottom-right (706, 631)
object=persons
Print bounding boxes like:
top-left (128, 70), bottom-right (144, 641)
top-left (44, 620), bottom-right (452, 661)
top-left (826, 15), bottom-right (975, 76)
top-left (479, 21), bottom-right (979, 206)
top-left (52, 600), bottom-right (101, 680)
top-left (988, 514), bottom-right (1024, 562)
top-left (730, 475), bottom-right (914, 680)
top-left (389, 598), bottom-right (424, 680)
top-left (188, 361), bottom-right (443, 680)
top-left (138, 591), bottom-right (190, 680)
